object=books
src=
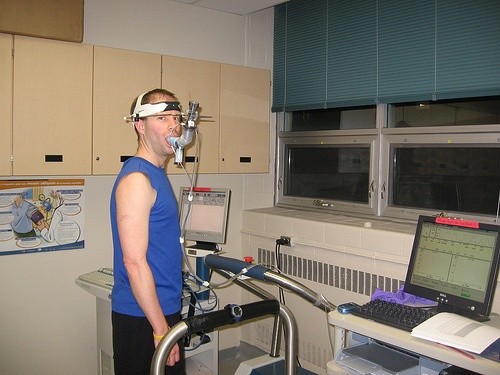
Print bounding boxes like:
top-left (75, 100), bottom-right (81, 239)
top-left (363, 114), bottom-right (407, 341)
top-left (410, 313), bottom-right (500, 354)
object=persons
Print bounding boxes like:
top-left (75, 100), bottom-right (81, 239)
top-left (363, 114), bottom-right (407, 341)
top-left (109, 90), bottom-right (182, 375)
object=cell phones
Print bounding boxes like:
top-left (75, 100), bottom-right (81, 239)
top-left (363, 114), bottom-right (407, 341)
top-left (338, 302), bottom-right (359, 314)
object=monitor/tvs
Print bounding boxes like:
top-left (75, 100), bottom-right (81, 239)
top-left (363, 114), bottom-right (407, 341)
top-left (403, 215), bottom-right (500, 322)
top-left (178, 186), bottom-right (231, 252)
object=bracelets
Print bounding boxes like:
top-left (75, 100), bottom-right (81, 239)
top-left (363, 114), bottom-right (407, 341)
top-left (153, 327), bottom-right (171, 340)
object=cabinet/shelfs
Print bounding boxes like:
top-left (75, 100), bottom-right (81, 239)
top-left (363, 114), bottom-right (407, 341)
top-left (327, 310), bottom-right (500, 375)
top-left (0, 33), bottom-right (271, 177)
top-left (75, 278), bottom-right (219, 375)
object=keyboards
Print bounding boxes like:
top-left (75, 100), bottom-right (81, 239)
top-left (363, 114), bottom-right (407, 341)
top-left (78, 268), bottom-right (116, 291)
top-left (351, 299), bottom-right (435, 332)
